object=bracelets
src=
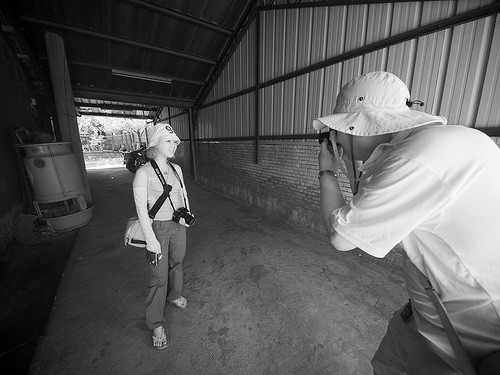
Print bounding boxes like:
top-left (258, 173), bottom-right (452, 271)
top-left (318, 170), bottom-right (338, 181)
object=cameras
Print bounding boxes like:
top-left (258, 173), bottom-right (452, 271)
top-left (318, 127), bottom-right (342, 145)
top-left (172, 206), bottom-right (195, 228)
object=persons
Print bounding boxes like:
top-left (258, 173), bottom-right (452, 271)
top-left (119, 144), bottom-right (127, 159)
top-left (312, 72), bottom-right (500, 375)
top-left (132, 124), bottom-right (194, 350)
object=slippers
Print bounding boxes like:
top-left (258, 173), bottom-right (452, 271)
top-left (152, 326), bottom-right (169, 349)
top-left (170, 296), bottom-right (188, 309)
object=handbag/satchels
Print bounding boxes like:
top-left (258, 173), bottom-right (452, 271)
top-left (124, 217), bottom-right (147, 248)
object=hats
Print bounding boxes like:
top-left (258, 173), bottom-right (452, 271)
top-left (313, 71), bottom-right (448, 136)
top-left (145, 123), bottom-right (181, 151)
